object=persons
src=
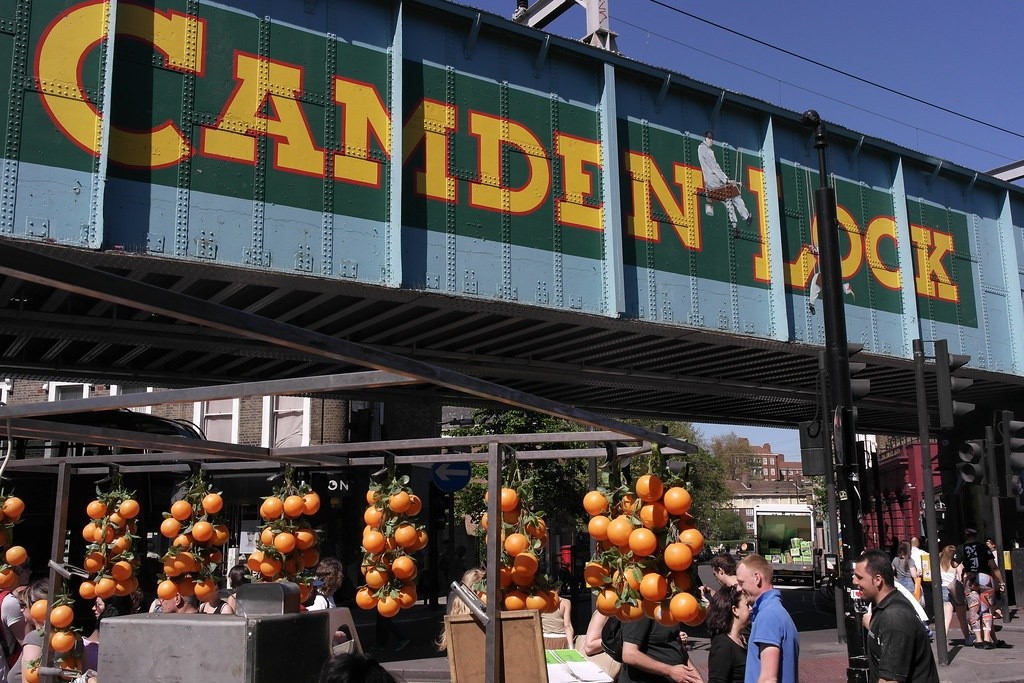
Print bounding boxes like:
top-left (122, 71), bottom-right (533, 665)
top-left (851, 529), bottom-right (1006, 683)
top-left (435, 544), bottom-right (799, 683)
top-left (0, 552), bottom-right (397, 683)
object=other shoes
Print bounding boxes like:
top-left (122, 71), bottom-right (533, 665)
top-left (993, 614), bottom-right (1000, 618)
top-left (995, 609), bottom-right (1003, 618)
top-left (965, 635), bottom-right (975, 646)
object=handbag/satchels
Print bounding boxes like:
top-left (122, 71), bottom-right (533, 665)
top-left (918, 585), bottom-right (925, 608)
top-left (947, 578), bottom-right (965, 606)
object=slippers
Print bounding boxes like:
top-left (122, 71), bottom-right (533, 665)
top-left (974, 637), bottom-right (995, 648)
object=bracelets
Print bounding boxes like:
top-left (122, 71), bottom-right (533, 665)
top-left (998, 583), bottom-right (1006, 586)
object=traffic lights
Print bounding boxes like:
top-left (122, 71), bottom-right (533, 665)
top-left (846, 342), bottom-right (872, 403)
top-left (959, 439), bottom-right (987, 486)
top-left (933, 339), bottom-right (975, 430)
top-left (1002, 410), bottom-right (1024, 498)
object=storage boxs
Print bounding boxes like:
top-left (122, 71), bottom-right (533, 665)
top-left (765, 537), bottom-right (811, 565)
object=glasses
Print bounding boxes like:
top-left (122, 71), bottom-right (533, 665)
top-left (95, 602), bottom-right (105, 607)
top-left (20, 603), bottom-right (27, 609)
top-left (730, 584), bottom-right (736, 596)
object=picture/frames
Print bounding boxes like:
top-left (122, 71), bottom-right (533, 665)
top-left (443, 608), bottom-right (549, 683)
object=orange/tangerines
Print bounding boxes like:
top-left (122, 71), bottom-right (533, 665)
top-left (248, 492), bottom-right (320, 603)
top-left (356, 491), bottom-right (428, 616)
top-left (26, 600), bottom-right (83, 683)
top-left (481, 488), bottom-right (559, 615)
top-left (156, 493), bottom-right (230, 602)
top-left (582, 474), bottom-right (707, 626)
top-left (0, 498), bottom-right (27, 589)
top-left (78, 498), bottom-right (138, 599)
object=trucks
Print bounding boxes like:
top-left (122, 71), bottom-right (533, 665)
top-left (753, 503), bottom-right (821, 588)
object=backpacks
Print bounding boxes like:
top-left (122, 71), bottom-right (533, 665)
top-left (0, 590), bottom-right (22, 670)
top-left (601, 615), bottom-right (626, 664)
